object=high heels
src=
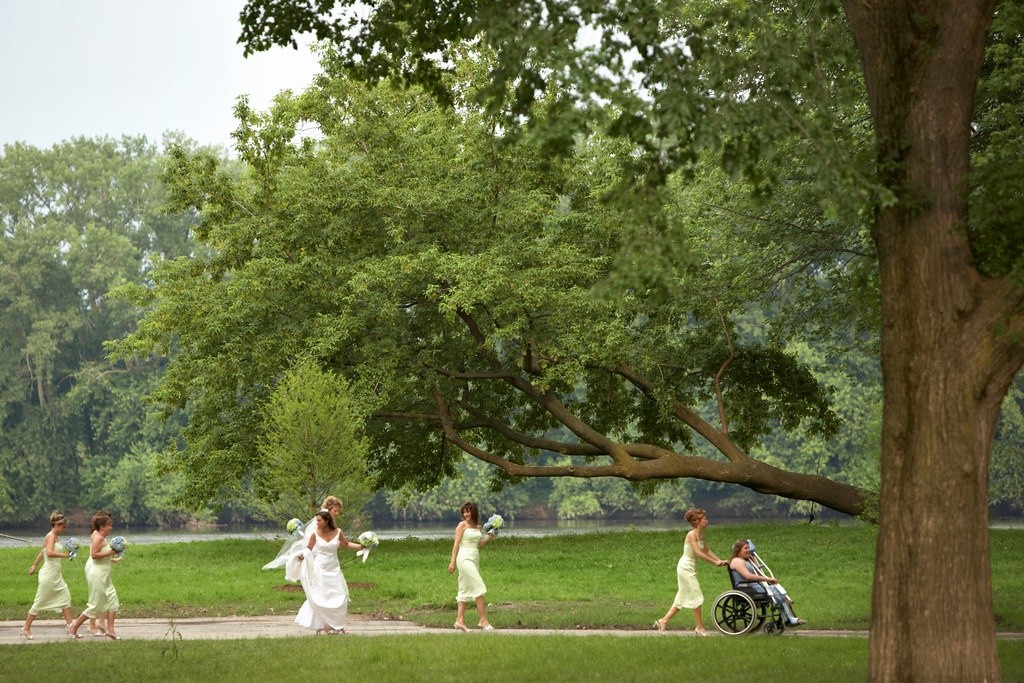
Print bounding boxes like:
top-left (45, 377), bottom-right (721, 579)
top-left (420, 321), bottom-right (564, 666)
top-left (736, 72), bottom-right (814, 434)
top-left (87, 626), bottom-right (106, 637)
top-left (104, 630), bottom-right (122, 641)
top-left (455, 623), bottom-right (472, 632)
top-left (653, 620), bottom-right (664, 634)
top-left (20, 628), bottom-right (36, 640)
top-left (695, 626), bottom-right (708, 636)
top-left (67, 627), bottom-right (83, 640)
top-left (476, 621), bottom-right (493, 631)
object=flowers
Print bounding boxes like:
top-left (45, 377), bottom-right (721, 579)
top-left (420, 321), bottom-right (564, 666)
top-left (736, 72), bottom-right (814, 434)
top-left (287, 518), bottom-right (304, 534)
top-left (359, 531), bottom-right (379, 549)
top-left (483, 514), bottom-right (505, 536)
top-left (110, 536), bottom-right (128, 558)
top-left (64, 537), bottom-right (82, 561)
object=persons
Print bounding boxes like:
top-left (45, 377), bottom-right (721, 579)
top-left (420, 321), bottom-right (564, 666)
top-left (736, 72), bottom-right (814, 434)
top-left (297, 511), bottom-right (365, 633)
top-left (86, 509), bottom-right (123, 636)
top-left (21, 510), bottom-right (83, 641)
top-left (64, 510), bottom-right (122, 641)
top-left (449, 499), bottom-right (495, 633)
top-left (730, 540), bottom-right (805, 625)
top-left (653, 508), bottom-right (728, 636)
top-left (304, 496), bottom-right (343, 635)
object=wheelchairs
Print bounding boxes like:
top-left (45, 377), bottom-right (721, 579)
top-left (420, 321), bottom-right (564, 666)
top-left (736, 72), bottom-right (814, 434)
top-left (709, 561), bottom-right (785, 637)
